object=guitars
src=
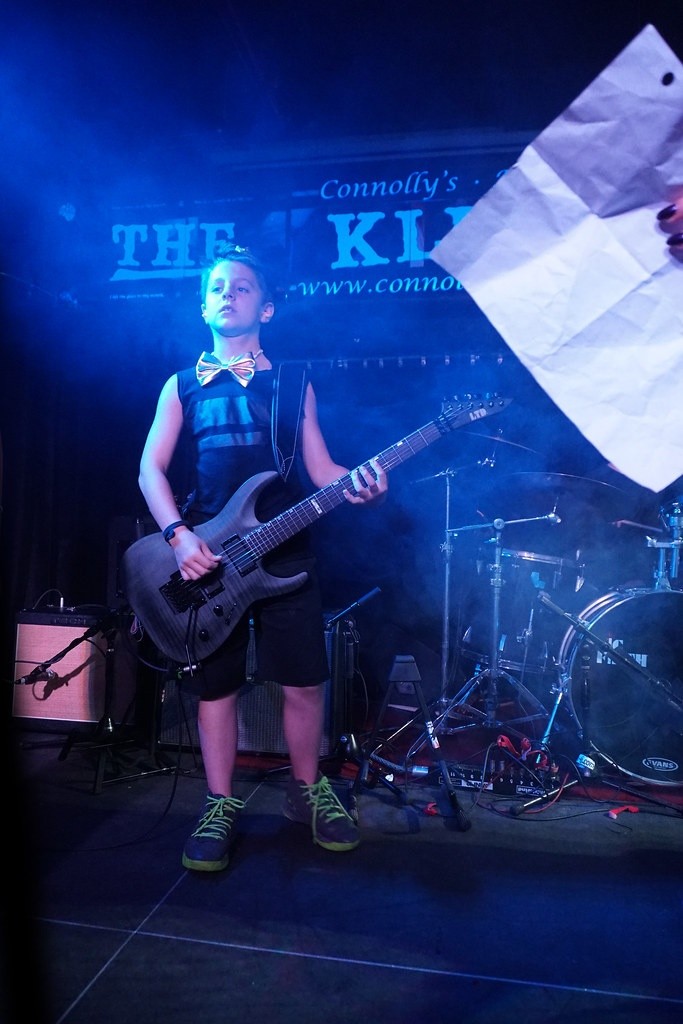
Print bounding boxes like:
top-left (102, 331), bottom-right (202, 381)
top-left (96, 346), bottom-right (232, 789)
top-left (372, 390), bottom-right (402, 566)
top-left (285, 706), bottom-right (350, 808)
top-left (119, 391), bottom-right (516, 670)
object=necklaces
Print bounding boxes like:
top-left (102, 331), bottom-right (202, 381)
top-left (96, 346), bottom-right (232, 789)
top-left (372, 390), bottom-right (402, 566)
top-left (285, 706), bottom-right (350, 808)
top-left (254, 350), bottom-right (263, 359)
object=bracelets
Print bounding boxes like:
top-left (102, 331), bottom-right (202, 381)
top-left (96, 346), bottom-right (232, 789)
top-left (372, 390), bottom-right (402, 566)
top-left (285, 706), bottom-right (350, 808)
top-left (162, 520), bottom-right (195, 546)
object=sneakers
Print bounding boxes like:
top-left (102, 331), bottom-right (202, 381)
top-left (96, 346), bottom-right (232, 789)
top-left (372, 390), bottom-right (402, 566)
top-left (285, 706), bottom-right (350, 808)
top-left (181, 788), bottom-right (247, 871)
top-left (280, 771), bottom-right (363, 851)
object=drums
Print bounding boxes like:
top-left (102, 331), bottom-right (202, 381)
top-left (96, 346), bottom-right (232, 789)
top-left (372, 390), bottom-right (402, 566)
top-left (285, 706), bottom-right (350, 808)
top-left (456, 531), bottom-right (583, 671)
top-left (555, 579), bottom-right (682, 789)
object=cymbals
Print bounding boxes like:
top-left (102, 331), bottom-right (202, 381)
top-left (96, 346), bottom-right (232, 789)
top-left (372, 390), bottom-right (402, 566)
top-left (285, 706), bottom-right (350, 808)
top-left (454, 419), bottom-right (539, 460)
top-left (512, 468), bottom-right (635, 517)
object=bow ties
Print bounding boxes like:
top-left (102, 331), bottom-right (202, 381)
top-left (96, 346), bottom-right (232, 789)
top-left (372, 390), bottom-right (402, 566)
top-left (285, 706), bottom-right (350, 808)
top-left (196, 350), bottom-right (263, 388)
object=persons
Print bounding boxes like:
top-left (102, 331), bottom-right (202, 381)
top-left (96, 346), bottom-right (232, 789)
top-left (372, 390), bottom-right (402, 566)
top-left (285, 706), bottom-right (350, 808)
top-left (138, 249), bottom-right (388, 871)
